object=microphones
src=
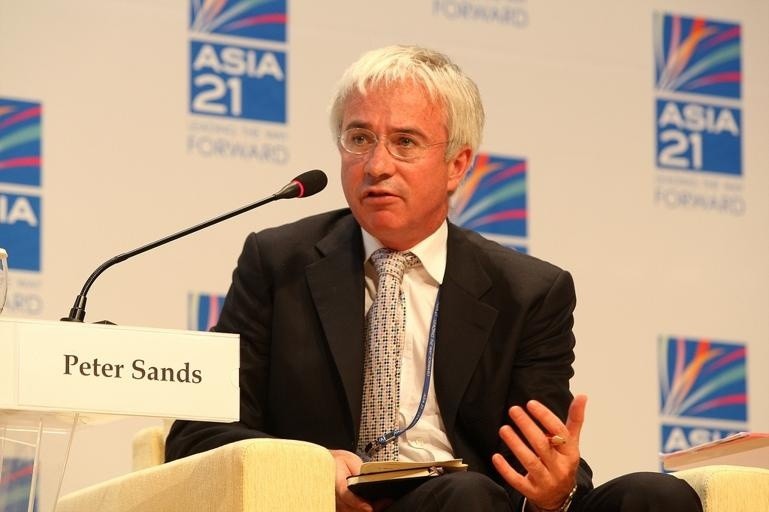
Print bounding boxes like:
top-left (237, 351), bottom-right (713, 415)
top-left (62, 169), bottom-right (327, 322)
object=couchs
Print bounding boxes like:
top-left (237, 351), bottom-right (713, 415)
top-left (56, 429), bottom-right (769, 512)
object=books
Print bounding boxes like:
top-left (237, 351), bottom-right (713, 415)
top-left (347, 467), bottom-right (440, 494)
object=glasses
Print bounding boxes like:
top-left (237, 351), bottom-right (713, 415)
top-left (337, 128), bottom-right (449, 160)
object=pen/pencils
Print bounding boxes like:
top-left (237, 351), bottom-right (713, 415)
top-left (544, 432), bottom-right (566, 447)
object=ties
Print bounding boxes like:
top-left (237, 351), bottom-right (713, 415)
top-left (354, 248), bottom-right (422, 463)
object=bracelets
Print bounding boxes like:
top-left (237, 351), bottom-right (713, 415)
top-left (559, 483), bottom-right (578, 512)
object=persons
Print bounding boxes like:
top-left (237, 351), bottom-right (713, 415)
top-left (164, 45), bottom-right (704, 512)
